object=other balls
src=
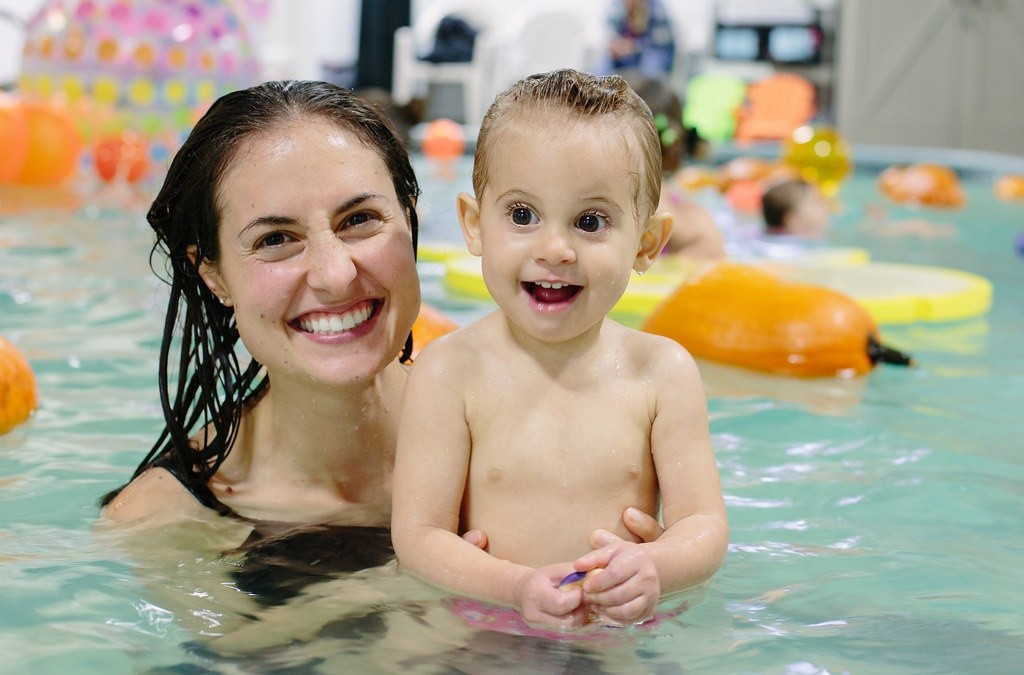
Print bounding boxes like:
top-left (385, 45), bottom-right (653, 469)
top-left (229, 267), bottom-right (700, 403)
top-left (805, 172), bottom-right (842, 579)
top-left (788, 122), bottom-right (849, 192)
top-left (20, 0), bottom-right (259, 211)
top-left (422, 117), bottom-right (463, 165)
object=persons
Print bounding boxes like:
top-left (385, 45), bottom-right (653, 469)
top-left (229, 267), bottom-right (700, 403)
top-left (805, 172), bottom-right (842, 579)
top-left (761, 179), bottom-right (825, 241)
top-left (389, 70), bottom-right (728, 637)
top-left (97, 80), bottom-right (488, 555)
top-left (633, 77), bottom-right (722, 274)
